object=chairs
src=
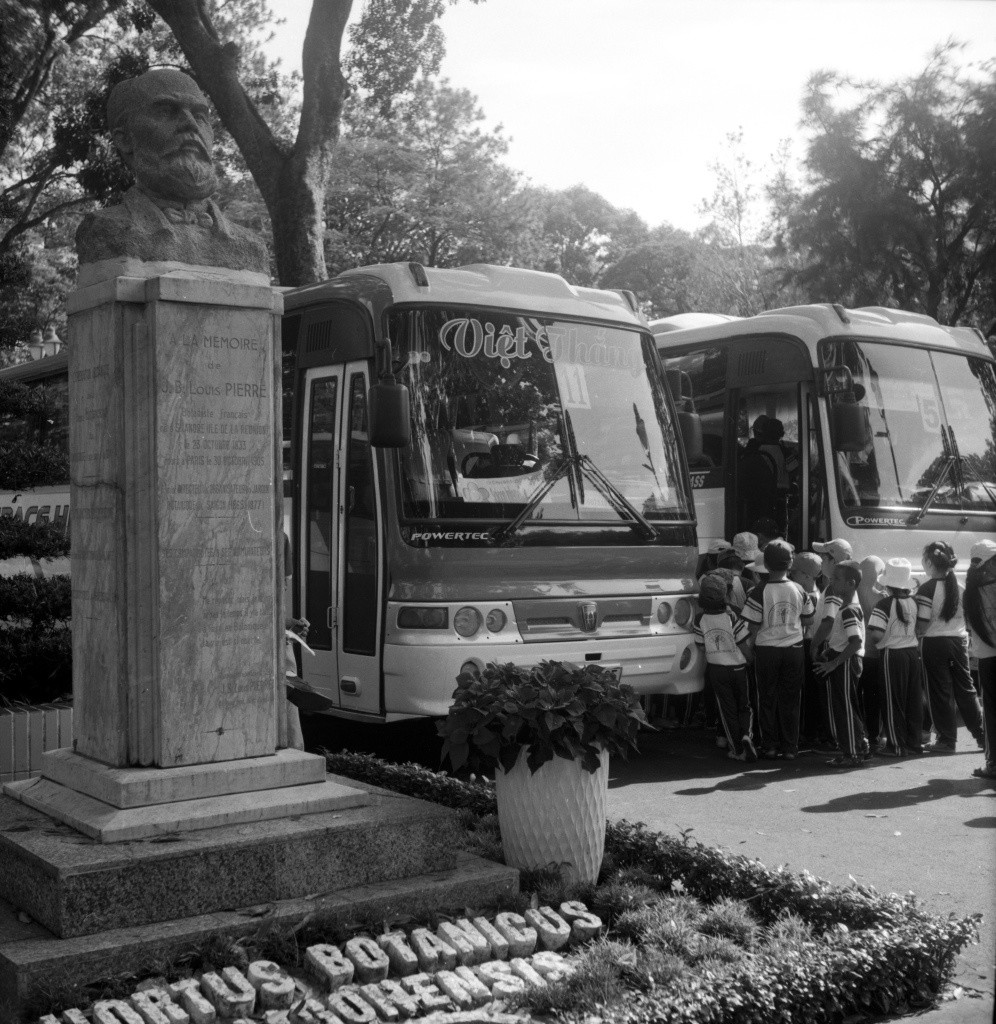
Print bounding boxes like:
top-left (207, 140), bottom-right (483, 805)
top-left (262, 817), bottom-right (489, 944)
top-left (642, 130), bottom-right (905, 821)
top-left (750, 437), bottom-right (801, 495)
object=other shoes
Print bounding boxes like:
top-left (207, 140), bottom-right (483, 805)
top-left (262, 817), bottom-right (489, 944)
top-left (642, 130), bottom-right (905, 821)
top-left (973, 767), bottom-right (995, 779)
top-left (716, 733), bottom-right (956, 766)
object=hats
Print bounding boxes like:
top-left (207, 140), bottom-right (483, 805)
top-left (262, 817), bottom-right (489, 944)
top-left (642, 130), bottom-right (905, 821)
top-left (707, 539), bottom-right (731, 553)
top-left (700, 575), bottom-right (727, 602)
top-left (732, 531), bottom-right (761, 561)
top-left (764, 540), bottom-right (793, 569)
top-left (876, 557), bottom-right (917, 589)
top-left (792, 552), bottom-right (823, 578)
top-left (812, 538), bottom-right (854, 562)
top-left (744, 553), bottom-right (769, 573)
top-left (750, 415), bottom-right (767, 433)
top-left (970, 538), bottom-right (996, 569)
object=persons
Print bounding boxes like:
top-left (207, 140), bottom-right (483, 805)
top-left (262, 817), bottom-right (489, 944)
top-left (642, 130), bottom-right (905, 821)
top-left (76, 69), bottom-right (270, 280)
top-left (692, 532), bottom-right (996, 781)
top-left (283, 531), bottom-right (334, 750)
top-left (739, 415), bottom-right (801, 530)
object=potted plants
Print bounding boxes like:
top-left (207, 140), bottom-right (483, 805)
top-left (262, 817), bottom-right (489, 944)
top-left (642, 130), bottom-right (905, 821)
top-left (434, 661), bottom-right (660, 887)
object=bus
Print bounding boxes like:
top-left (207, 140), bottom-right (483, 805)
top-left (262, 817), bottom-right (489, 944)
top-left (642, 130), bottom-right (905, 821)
top-left (273, 259), bottom-right (716, 744)
top-left (643, 300), bottom-right (996, 682)
